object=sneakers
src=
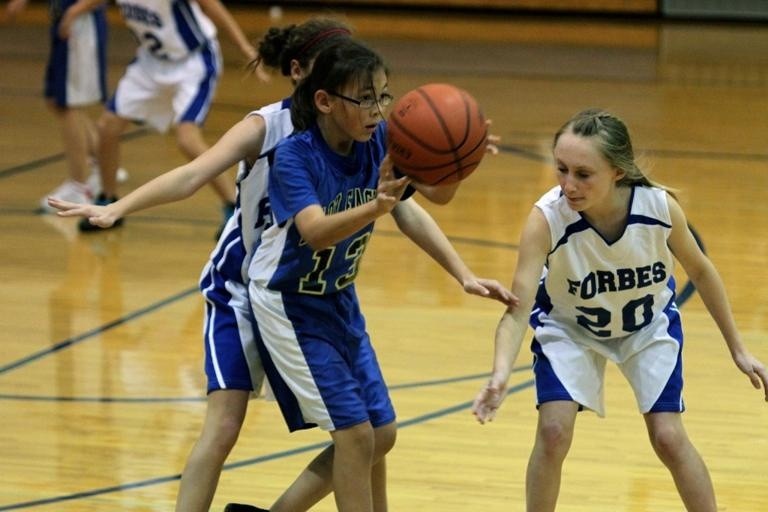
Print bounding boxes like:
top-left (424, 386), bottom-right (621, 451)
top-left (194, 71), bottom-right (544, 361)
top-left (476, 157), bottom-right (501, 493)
top-left (81, 192), bottom-right (122, 231)
top-left (43, 174), bottom-right (99, 213)
top-left (216, 200), bottom-right (236, 239)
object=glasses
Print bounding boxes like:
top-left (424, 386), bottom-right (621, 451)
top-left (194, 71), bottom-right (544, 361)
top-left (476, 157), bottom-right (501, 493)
top-left (332, 91), bottom-right (394, 110)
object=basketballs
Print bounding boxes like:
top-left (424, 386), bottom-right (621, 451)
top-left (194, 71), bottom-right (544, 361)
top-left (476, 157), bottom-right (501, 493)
top-left (387, 82), bottom-right (489, 186)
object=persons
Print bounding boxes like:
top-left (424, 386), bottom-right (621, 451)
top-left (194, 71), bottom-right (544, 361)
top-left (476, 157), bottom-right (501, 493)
top-left (1, 0), bottom-right (114, 215)
top-left (473, 106), bottom-right (767, 512)
top-left (40, 11), bottom-right (521, 512)
top-left (58, 0), bottom-right (270, 240)
top-left (227, 38), bottom-right (502, 512)
